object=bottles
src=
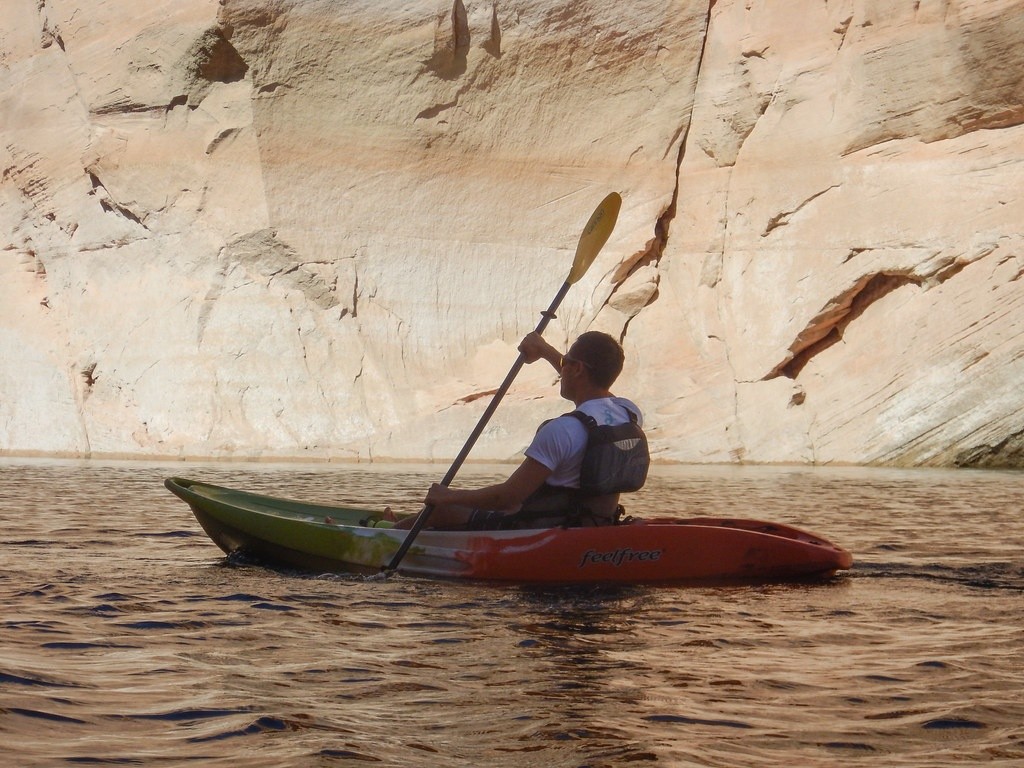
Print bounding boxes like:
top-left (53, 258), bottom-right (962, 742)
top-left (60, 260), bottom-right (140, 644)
top-left (359, 515), bottom-right (395, 529)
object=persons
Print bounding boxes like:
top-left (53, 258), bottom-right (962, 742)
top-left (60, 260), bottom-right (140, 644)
top-left (325, 329), bottom-right (650, 530)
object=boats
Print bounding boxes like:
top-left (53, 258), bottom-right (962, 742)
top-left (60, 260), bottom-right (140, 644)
top-left (162, 479), bottom-right (857, 595)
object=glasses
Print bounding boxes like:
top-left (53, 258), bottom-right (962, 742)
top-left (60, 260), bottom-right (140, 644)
top-left (559, 354), bottom-right (593, 370)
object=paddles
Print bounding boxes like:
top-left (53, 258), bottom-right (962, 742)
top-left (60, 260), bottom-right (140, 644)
top-left (379, 189), bottom-right (624, 578)
